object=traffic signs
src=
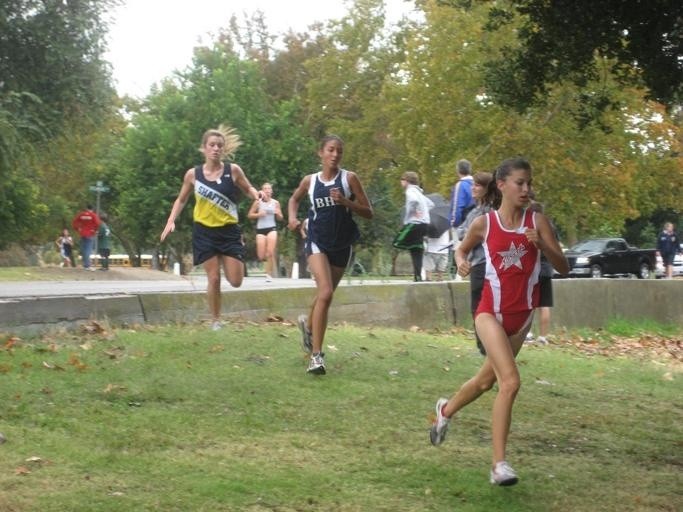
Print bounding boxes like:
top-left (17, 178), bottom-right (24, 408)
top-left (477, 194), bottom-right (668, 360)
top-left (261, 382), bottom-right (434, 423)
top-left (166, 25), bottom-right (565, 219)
top-left (88, 186), bottom-right (110, 193)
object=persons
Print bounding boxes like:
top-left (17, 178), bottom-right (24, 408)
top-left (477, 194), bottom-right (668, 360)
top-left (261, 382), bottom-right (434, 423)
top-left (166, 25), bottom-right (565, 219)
top-left (523, 191), bottom-right (559, 345)
top-left (300, 216), bottom-right (317, 280)
top-left (655, 222), bottom-right (682, 280)
top-left (428, 158), bottom-right (572, 488)
top-left (447, 158), bottom-right (478, 281)
top-left (422, 227), bottom-right (448, 282)
top-left (287, 134), bottom-right (375, 377)
top-left (160, 123), bottom-right (267, 332)
top-left (54, 228), bottom-right (74, 268)
top-left (96, 217), bottom-right (112, 272)
top-left (454, 171), bottom-right (493, 356)
top-left (245, 181), bottom-right (285, 284)
top-left (391, 171), bottom-right (435, 283)
top-left (71, 203), bottom-right (101, 272)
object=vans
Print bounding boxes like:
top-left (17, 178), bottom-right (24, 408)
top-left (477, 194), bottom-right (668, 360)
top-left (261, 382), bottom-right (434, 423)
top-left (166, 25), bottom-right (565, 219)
top-left (655, 243), bottom-right (683, 275)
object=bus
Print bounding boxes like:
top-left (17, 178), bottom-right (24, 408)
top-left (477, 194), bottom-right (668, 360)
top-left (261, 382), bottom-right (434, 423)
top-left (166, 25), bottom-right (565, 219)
top-left (73, 254), bottom-right (168, 272)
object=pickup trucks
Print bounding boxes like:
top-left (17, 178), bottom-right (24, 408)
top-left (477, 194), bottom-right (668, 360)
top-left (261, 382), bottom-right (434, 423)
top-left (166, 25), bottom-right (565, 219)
top-left (550, 237), bottom-right (659, 280)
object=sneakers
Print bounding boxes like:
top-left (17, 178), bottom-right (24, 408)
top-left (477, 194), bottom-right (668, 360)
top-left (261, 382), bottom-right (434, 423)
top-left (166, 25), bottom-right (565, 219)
top-left (296, 314), bottom-right (313, 352)
top-left (489, 460), bottom-right (518, 486)
top-left (307, 351), bottom-right (326, 375)
top-left (212, 320), bottom-right (223, 331)
top-left (430, 397), bottom-right (450, 446)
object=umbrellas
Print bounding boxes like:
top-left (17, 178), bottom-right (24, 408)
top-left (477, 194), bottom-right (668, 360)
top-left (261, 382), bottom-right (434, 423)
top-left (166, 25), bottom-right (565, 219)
top-left (399, 192), bottom-right (451, 239)
